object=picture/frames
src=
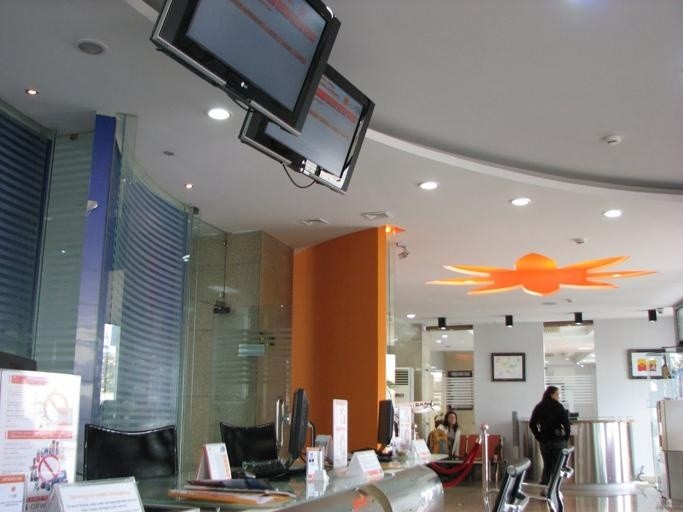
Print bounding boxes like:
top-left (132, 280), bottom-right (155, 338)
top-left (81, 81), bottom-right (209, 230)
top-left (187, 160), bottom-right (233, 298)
top-left (628, 349), bottom-right (671, 379)
top-left (490, 353), bottom-right (525, 381)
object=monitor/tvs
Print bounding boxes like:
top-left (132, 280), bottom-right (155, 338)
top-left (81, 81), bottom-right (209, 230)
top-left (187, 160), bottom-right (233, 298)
top-left (149, 0), bottom-right (342, 136)
top-left (377, 399), bottom-right (394, 444)
top-left (289, 388), bottom-right (309, 456)
top-left (237, 60), bottom-right (376, 195)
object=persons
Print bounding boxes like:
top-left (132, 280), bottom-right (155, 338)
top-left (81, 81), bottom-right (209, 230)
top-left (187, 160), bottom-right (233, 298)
top-left (437, 411), bottom-right (460, 456)
top-left (529, 386), bottom-right (570, 485)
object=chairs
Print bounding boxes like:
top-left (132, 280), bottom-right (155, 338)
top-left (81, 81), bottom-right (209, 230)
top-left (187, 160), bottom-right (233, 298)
top-left (428, 433), bottom-right (505, 492)
top-left (83, 423), bottom-right (180, 484)
top-left (218, 418), bottom-right (279, 473)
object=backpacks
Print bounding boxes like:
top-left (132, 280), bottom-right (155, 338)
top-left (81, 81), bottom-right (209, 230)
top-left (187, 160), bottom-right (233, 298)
top-left (427, 429), bottom-right (449, 455)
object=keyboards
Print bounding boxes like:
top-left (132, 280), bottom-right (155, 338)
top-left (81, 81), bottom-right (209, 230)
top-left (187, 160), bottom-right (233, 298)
top-left (245, 458), bottom-right (284, 477)
top-left (351, 447), bottom-right (390, 462)
